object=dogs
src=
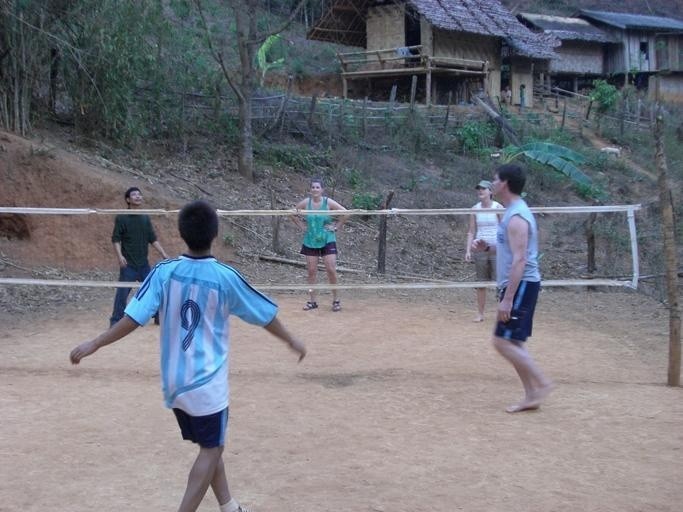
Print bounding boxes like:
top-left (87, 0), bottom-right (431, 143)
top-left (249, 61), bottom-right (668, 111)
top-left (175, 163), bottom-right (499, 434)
top-left (600, 147), bottom-right (622, 159)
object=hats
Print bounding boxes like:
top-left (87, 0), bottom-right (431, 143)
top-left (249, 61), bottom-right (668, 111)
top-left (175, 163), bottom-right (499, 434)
top-left (475, 180), bottom-right (492, 190)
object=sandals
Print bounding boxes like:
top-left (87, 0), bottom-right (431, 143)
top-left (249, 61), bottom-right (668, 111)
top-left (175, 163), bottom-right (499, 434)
top-left (332, 299), bottom-right (341, 312)
top-left (303, 301), bottom-right (318, 310)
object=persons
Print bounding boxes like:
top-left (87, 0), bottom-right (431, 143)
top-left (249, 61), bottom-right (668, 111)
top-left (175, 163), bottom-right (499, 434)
top-left (463, 179), bottom-right (505, 322)
top-left (471, 163), bottom-right (555, 417)
top-left (286, 181), bottom-right (350, 312)
top-left (67, 199), bottom-right (306, 512)
top-left (108, 186), bottom-right (171, 326)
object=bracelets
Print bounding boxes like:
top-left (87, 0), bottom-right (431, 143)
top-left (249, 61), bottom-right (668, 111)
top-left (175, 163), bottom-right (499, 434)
top-left (483, 242), bottom-right (491, 252)
top-left (333, 226), bottom-right (337, 232)
